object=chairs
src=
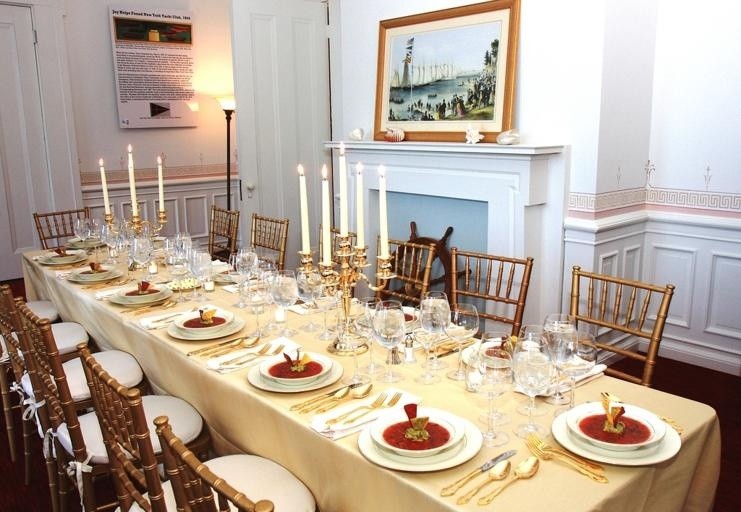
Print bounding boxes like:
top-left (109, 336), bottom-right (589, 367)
top-left (0, 284), bottom-right (58, 326)
top-left (33, 207), bottom-right (92, 249)
top-left (0, 295), bottom-right (89, 460)
top-left (373, 234), bottom-right (436, 311)
top-left (251, 212), bottom-right (289, 272)
top-left (447, 244), bottom-right (532, 345)
top-left (10, 296), bottom-right (206, 512)
top-left (208, 205), bottom-right (240, 263)
top-left (560, 264), bottom-right (674, 387)
top-left (7, 295), bottom-right (143, 486)
top-left (75, 342), bottom-right (316, 512)
top-left (154, 415), bottom-right (268, 509)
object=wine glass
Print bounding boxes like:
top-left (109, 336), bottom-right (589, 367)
top-left (236, 248), bottom-right (257, 310)
top-left (165, 231), bottom-right (212, 276)
top-left (73, 217), bottom-right (105, 238)
top-left (415, 307), bottom-right (443, 385)
top-left (228, 253), bottom-right (247, 307)
top-left (544, 312), bottom-right (578, 406)
top-left (421, 291), bottom-right (451, 369)
top-left (242, 278), bottom-right (270, 339)
top-left (445, 303), bottom-right (479, 381)
top-left (337, 308), bottom-right (373, 386)
top-left (105, 222), bottom-right (157, 265)
top-left (272, 270), bottom-right (299, 338)
top-left (311, 282), bottom-right (338, 339)
top-left (519, 325), bottom-right (550, 349)
top-left (257, 264), bottom-right (273, 280)
top-left (372, 300), bottom-right (405, 383)
top-left (297, 270), bottom-right (319, 333)
top-left (466, 349), bottom-right (511, 448)
top-left (512, 337), bottom-right (554, 441)
top-left (358, 296), bottom-right (387, 376)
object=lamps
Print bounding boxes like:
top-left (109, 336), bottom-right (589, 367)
top-left (213, 100), bottom-right (241, 263)
top-left (355, 162), bottom-right (366, 249)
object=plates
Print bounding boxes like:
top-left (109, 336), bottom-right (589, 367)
top-left (552, 408), bottom-right (682, 466)
top-left (175, 307), bottom-right (234, 333)
top-left (167, 316), bottom-right (247, 340)
top-left (402, 307), bottom-right (419, 324)
top-left (210, 274), bottom-right (240, 286)
top-left (120, 284), bottom-right (167, 300)
top-left (49, 251), bottom-right (80, 262)
top-left (74, 266), bottom-right (112, 279)
top-left (370, 408), bottom-right (466, 458)
top-left (39, 257), bottom-right (88, 266)
top-left (69, 238), bottom-right (102, 246)
top-left (67, 270), bottom-right (124, 284)
top-left (480, 341), bottom-right (512, 361)
top-left (567, 402), bottom-right (666, 452)
top-left (259, 351), bottom-right (333, 385)
top-left (167, 282), bottom-right (202, 292)
top-left (462, 337), bottom-right (501, 368)
top-left (247, 359), bottom-right (343, 393)
top-left (358, 416), bottom-right (483, 472)
top-left (64, 243), bottom-right (105, 248)
top-left (109, 290), bottom-right (173, 307)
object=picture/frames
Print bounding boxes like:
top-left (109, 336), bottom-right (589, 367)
top-left (373, 0), bottom-right (520, 146)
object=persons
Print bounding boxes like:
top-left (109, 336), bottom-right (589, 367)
top-left (388, 69), bottom-right (495, 120)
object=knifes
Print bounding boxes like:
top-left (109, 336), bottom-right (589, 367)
top-left (441, 449), bottom-right (517, 496)
top-left (430, 341), bottom-right (474, 360)
top-left (291, 383), bottom-right (362, 411)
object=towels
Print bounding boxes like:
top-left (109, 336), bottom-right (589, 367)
top-left (208, 257), bottom-right (227, 266)
top-left (140, 303), bottom-right (217, 329)
top-left (208, 336), bottom-right (298, 373)
top-left (509, 354), bottom-right (607, 397)
top-left (223, 281), bottom-right (254, 296)
top-left (94, 285), bottom-right (124, 300)
top-left (290, 297), bottom-right (336, 315)
top-left (54, 267), bottom-right (99, 277)
top-left (310, 389), bottom-right (422, 440)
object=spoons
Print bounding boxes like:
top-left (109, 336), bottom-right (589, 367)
top-left (134, 300), bottom-right (178, 317)
top-left (87, 277), bottom-right (128, 291)
top-left (200, 339), bottom-right (242, 358)
top-left (455, 460), bottom-right (511, 506)
top-left (211, 336), bottom-right (260, 358)
top-left (299, 387), bottom-right (352, 414)
top-left (316, 384), bottom-right (373, 414)
top-left (478, 455), bottom-right (540, 506)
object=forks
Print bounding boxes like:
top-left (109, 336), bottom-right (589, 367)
top-left (219, 342), bottom-right (273, 366)
top-left (152, 306), bottom-right (200, 323)
top-left (238, 345), bottom-right (285, 366)
top-left (527, 442), bottom-right (608, 483)
top-left (527, 431), bottom-right (606, 474)
top-left (325, 393), bottom-right (389, 424)
top-left (343, 392), bottom-right (403, 422)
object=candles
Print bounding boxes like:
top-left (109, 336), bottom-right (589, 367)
top-left (127, 144), bottom-right (139, 217)
top-left (338, 142), bottom-right (349, 237)
top-left (322, 165), bottom-right (333, 265)
top-left (99, 158), bottom-right (112, 215)
top-left (297, 164), bottom-right (312, 253)
top-left (157, 156), bottom-right (166, 212)
top-left (377, 163), bottom-right (391, 257)
